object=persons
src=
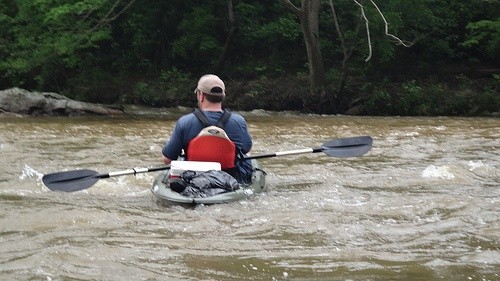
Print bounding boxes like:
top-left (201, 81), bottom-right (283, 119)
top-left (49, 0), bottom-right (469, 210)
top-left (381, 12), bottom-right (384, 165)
top-left (161, 74), bottom-right (253, 185)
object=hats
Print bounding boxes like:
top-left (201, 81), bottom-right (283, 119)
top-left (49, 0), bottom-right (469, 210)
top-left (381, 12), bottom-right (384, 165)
top-left (192, 75), bottom-right (226, 96)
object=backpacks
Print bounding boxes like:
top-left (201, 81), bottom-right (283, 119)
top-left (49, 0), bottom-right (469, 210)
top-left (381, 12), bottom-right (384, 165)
top-left (187, 109), bottom-right (236, 169)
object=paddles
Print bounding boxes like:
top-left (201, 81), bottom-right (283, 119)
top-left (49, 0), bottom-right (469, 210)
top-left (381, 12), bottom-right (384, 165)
top-left (42, 136), bottom-right (373, 193)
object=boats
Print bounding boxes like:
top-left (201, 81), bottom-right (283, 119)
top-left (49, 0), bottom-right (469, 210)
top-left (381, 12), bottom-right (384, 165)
top-left (151, 153), bottom-right (267, 210)
